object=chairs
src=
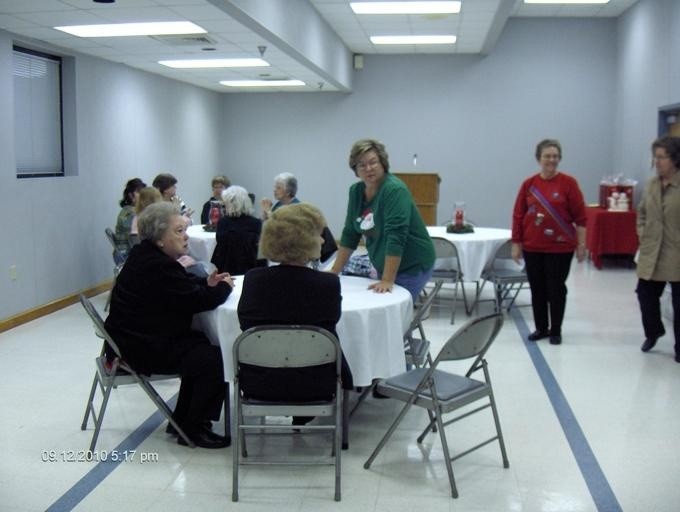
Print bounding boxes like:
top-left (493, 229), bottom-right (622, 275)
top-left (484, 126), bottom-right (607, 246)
top-left (79, 292), bottom-right (196, 461)
top-left (230, 323), bottom-right (349, 502)
top-left (469, 237), bottom-right (530, 315)
top-left (412, 236), bottom-right (470, 324)
top-left (346, 280), bottom-right (445, 432)
top-left (364, 312), bottom-right (510, 498)
top-left (103, 228), bottom-right (126, 312)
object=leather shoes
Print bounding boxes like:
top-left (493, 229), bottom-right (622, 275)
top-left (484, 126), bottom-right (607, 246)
top-left (641, 328), bottom-right (666, 353)
top-left (166, 417), bottom-right (232, 448)
top-left (527, 328), bottom-right (551, 341)
top-left (549, 330), bottom-right (561, 345)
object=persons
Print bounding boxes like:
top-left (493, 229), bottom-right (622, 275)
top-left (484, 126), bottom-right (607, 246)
top-left (112, 177), bottom-right (147, 265)
top-left (211, 185), bottom-right (267, 275)
top-left (103, 202), bottom-right (236, 449)
top-left (512, 139), bottom-right (587, 345)
top-left (201, 175), bottom-right (233, 224)
top-left (131, 186), bottom-right (167, 250)
top-left (327, 138), bottom-right (437, 399)
top-left (151, 173), bottom-right (192, 226)
top-left (635, 136), bottom-right (680, 363)
top-left (236, 203), bottom-right (353, 427)
top-left (261, 172), bottom-right (300, 219)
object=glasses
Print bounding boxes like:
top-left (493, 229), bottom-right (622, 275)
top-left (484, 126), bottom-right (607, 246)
top-left (357, 159), bottom-right (380, 171)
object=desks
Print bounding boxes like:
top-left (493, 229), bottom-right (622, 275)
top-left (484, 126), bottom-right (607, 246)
top-left (586, 204), bottom-right (638, 270)
top-left (184, 222), bottom-right (279, 271)
top-left (197, 270), bottom-right (411, 448)
top-left (424, 224), bottom-right (512, 309)
